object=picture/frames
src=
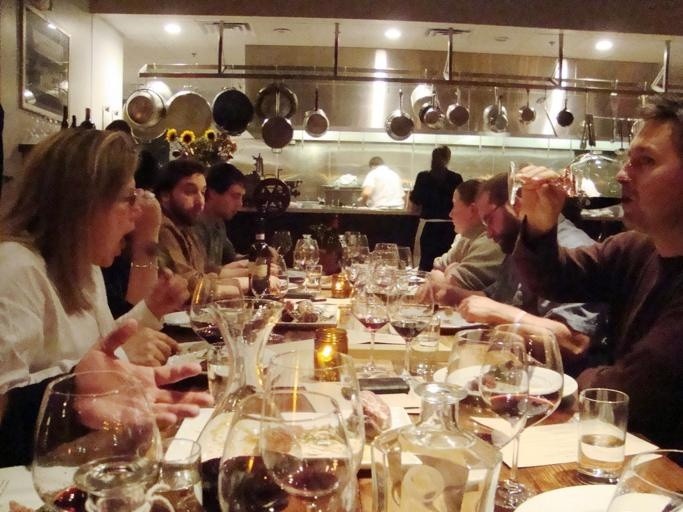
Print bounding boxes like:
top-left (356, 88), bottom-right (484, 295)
top-left (20, 3), bottom-right (70, 124)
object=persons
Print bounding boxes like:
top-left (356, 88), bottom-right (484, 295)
top-left (192, 163), bottom-right (251, 277)
top-left (358, 156), bottom-right (406, 209)
top-left (0, 319), bottom-right (216, 469)
top-left (93, 188), bottom-right (162, 321)
top-left (512, 93), bottom-right (683, 469)
top-left (415, 172), bottom-right (625, 381)
top-left (408, 144), bottom-right (464, 272)
top-left (148, 160), bottom-right (252, 304)
top-left (0, 129), bottom-right (191, 395)
top-left (428, 179), bottom-right (506, 291)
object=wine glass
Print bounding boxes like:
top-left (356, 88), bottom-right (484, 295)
top-left (31, 215), bottom-right (683, 512)
top-left (507, 153), bottom-right (631, 209)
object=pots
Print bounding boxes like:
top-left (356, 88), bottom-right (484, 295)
top-left (121, 78), bottom-right (574, 154)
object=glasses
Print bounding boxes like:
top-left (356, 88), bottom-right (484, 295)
top-left (481, 203), bottom-right (504, 226)
top-left (117, 187), bottom-right (138, 208)
